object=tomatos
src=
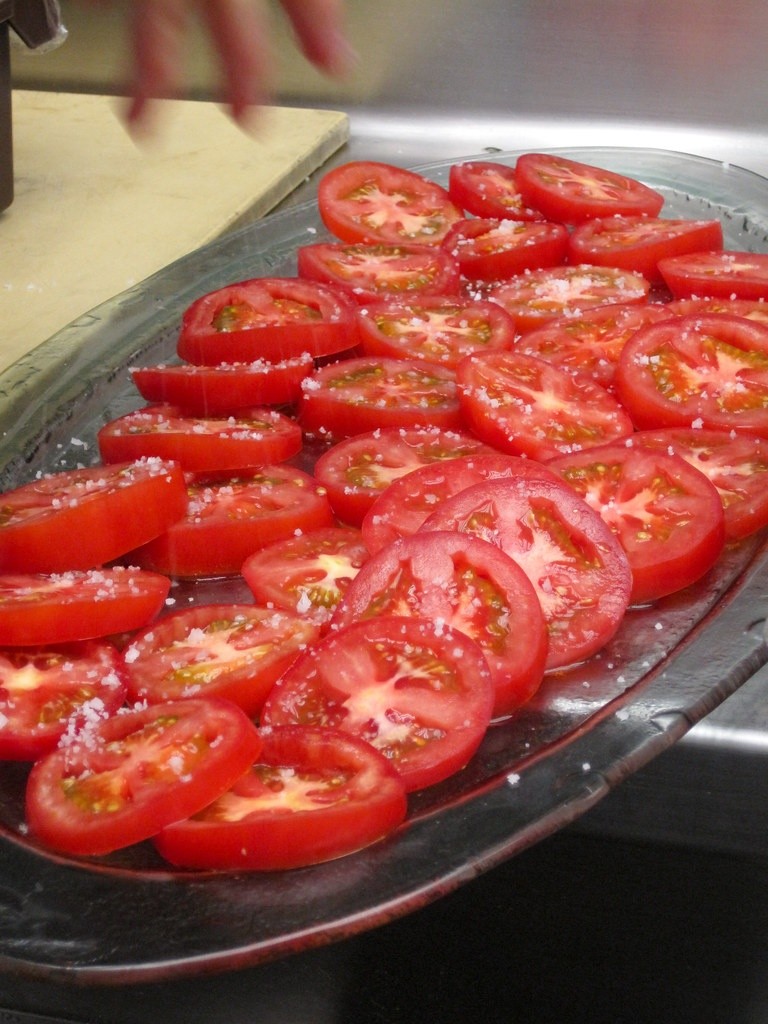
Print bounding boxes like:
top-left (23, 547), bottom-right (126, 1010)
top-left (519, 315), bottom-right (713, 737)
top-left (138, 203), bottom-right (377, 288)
top-left (0, 151), bottom-right (768, 873)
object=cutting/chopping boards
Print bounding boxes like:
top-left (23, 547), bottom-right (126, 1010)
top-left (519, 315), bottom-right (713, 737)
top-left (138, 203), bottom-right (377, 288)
top-left (0, 82), bottom-right (350, 481)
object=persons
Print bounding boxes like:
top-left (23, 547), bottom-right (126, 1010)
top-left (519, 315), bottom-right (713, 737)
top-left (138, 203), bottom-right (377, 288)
top-left (105, 1), bottom-right (356, 143)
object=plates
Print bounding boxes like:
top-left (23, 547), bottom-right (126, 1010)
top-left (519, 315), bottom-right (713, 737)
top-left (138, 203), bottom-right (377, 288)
top-left (0, 149), bottom-right (765, 990)
